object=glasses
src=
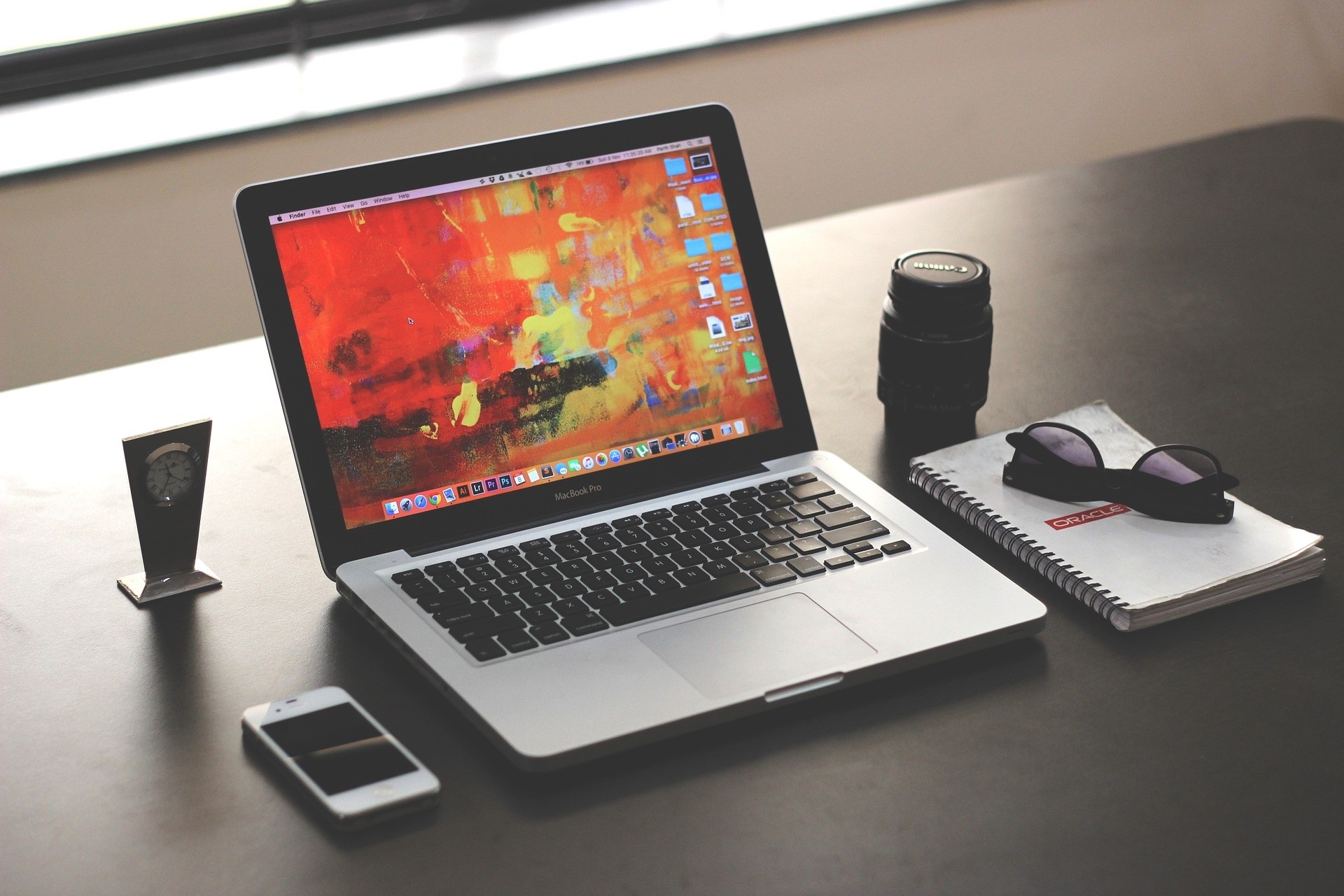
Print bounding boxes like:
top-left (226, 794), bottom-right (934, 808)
top-left (999, 419), bottom-right (1241, 528)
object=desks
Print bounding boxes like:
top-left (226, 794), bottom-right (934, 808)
top-left (0, 114), bottom-right (1344, 896)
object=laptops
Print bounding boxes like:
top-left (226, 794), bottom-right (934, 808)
top-left (230, 103), bottom-right (1049, 774)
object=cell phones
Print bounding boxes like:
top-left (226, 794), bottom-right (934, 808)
top-left (240, 684), bottom-right (442, 831)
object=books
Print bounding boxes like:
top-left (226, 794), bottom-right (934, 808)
top-left (907, 397), bottom-right (1327, 637)
